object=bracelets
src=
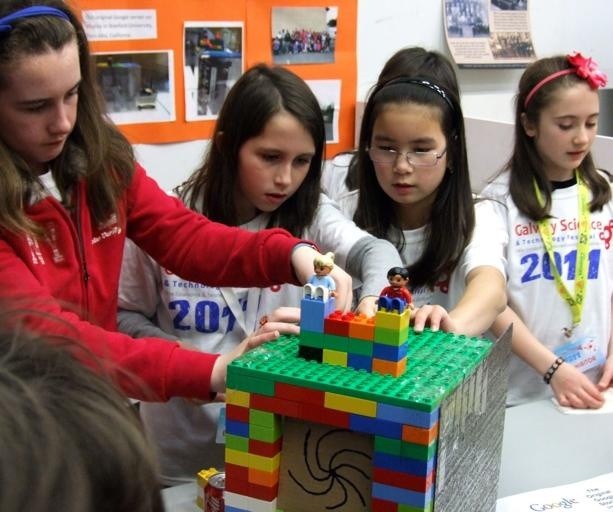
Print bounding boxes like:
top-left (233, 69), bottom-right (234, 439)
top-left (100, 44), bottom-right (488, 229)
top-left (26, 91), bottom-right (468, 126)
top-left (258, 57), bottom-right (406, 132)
top-left (543, 357), bottom-right (565, 385)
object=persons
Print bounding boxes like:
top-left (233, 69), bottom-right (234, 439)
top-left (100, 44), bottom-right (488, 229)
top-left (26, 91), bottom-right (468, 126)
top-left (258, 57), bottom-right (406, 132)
top-left (1, 307), bottom-right (165, 512)
top-left (195, 30), bottom-right (225, 55)
top-left (303, 252), bottom-right (337, 302)
top-left (318, 48), bottom-right (460, 198)
top-left (446, 1), bottom-right (534, 57)
top-left (331, 78), bottom-right (505, 335)
top-left (375, 266), bottom-right (413, 315)
top-left (1, 0), bottom-right (352, 403)
top-left (272, 28), bottom-right (336, 56)
top-left (481, 59), bottom-right (613, 409)
top-left (118, 64), bottom-right (406, 489)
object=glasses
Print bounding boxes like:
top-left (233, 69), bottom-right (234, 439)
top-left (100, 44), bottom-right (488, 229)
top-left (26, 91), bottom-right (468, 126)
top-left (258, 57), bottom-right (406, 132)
top-left (365, 127), bottom-right (456, 169)
